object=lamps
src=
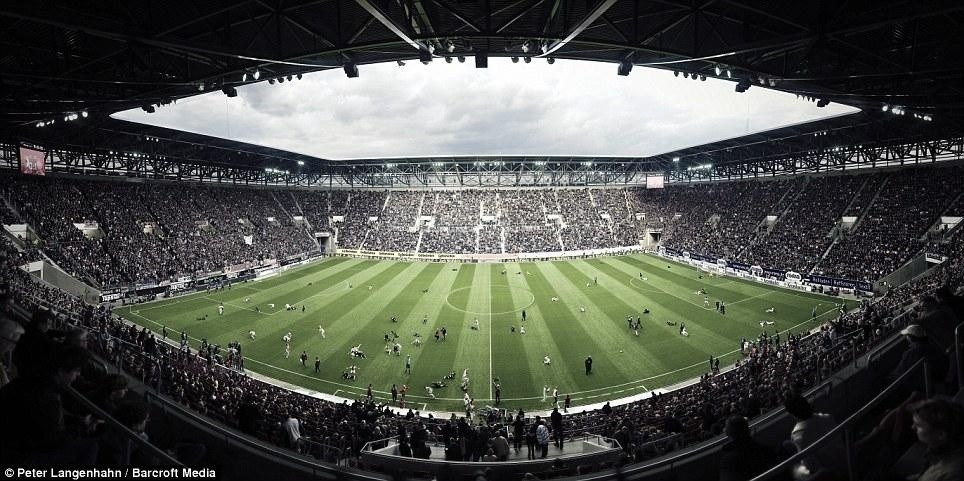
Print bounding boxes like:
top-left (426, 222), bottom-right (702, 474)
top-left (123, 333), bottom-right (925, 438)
top-left (139, 34), bottom-right (832, 114)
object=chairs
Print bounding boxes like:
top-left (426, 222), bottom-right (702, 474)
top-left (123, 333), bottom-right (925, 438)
top-left (2, 269), bottom-right (964, 480)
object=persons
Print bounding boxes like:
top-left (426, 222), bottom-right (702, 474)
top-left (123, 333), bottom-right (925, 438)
top-left (0, 165), bottom-right (964, 481)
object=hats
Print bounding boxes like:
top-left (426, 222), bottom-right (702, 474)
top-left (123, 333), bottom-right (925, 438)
top-left (900, 325), bottom-right (925, 336)
